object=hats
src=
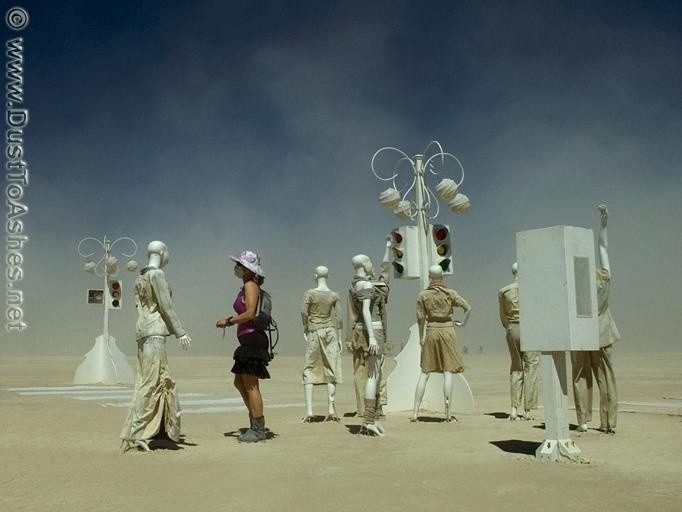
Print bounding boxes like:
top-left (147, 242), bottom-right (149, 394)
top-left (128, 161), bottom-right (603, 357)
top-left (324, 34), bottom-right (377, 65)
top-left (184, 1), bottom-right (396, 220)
top-left (229, 250), bottom-right (266, 278)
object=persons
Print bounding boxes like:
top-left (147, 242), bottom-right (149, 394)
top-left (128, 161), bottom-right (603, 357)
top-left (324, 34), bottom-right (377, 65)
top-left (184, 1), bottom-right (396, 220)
top-left (345, 253), bottom-right (388, 438)
top-left (300, 266), bottom-right (343, 423)
top-left (571, 203), bottom-right (621, 434)
top-left (216, 249), bottom-right (270, 442)
top-left (410, 265), bottom-right (472, 423)
top-left (346, 242), bottom-right (393, 416)
top-left (498, 262), bottom-right (541, 421)
top-left (120, 239), bottom-right (193, 453)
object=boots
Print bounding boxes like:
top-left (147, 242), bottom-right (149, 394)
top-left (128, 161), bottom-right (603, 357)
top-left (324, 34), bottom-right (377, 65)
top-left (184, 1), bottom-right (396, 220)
top-left (238, 415), bottom-right (267, 442)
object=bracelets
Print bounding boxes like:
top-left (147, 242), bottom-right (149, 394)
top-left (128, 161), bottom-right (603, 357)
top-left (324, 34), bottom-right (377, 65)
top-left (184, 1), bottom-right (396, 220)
top-left (225, 319), bottom-right (229, 326)
top-left (228, 316), bottom-right (234, 326)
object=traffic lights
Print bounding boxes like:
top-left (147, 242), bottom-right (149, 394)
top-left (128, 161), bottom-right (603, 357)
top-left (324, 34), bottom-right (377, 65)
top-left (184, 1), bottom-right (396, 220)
top-left (108, 276), bottom-right (122, 311)
top-left (389, 225), bottom-right (420, 281)
top-left (86, 288), bottom-right (105, 304)
top-left (425, 221), bottom-right (454, 278)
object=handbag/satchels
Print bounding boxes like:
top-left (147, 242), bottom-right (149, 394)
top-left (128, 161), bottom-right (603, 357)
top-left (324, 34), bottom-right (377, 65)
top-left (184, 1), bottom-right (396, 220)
top-left (252, 288), bottom-right (272, 332)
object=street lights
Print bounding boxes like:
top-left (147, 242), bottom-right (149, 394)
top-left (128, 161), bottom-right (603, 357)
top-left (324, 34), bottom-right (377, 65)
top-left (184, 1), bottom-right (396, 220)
top-left (71, 233), bottom-right (138, 389)
top-left (369, 137), bottom-right (478, 416)
